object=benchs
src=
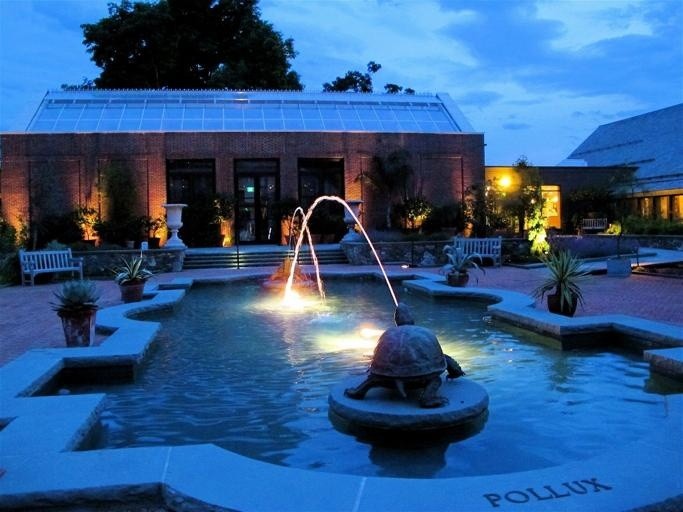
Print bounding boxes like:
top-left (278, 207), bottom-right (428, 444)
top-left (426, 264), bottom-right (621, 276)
top-left (18, 247), bottom-right (84, 287)
top-left (581, 218), bottom-right (608, 234)
top-left (447, 237), bottom-right (503, 268)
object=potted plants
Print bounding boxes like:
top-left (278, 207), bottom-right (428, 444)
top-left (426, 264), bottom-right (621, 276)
top-left (103, 249), bottom-right (161, 303)
top-left (437, 233), bottom-right (486, 286)
top-left (526, 246), bottom-right (594, 317)
top-left (47, 279), bottom-right (105, 347)
